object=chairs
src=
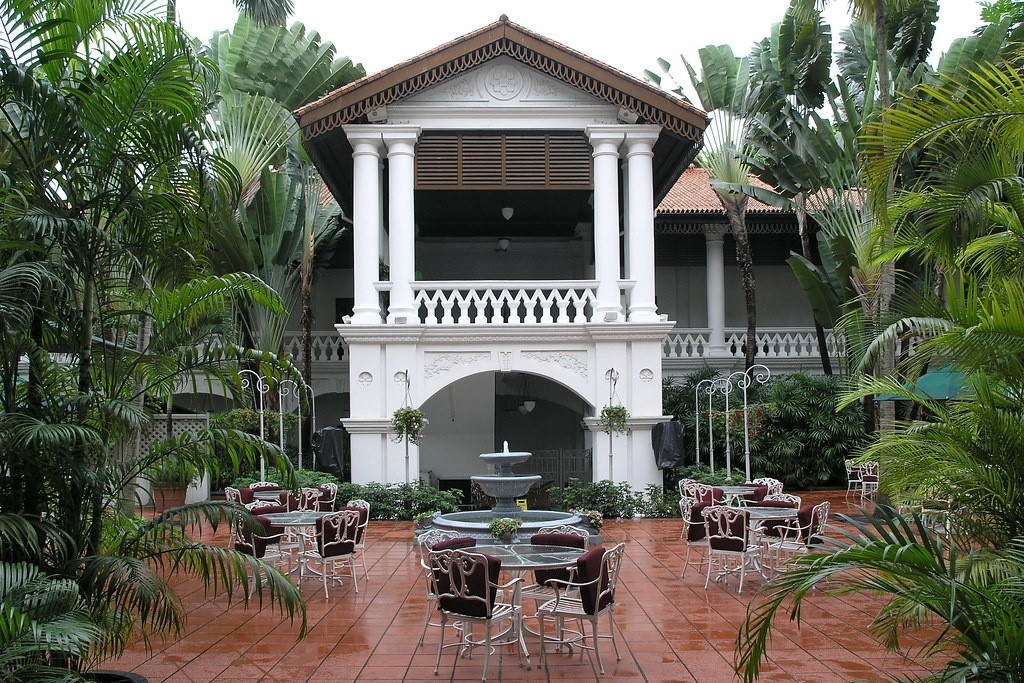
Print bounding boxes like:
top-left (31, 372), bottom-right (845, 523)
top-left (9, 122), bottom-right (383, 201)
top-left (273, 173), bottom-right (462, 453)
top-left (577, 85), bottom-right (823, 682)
top-left (419, 525), bottom-right (628, 683)
top-left (224, 479), bottom-right (370, 599)
top-left (844, 458), bottom-right (989, 539)
top-left (678, 477), bottom-right (831, 596)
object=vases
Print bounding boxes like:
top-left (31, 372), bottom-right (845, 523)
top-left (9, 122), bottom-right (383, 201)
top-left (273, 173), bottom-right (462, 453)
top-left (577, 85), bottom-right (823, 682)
top-left (498, 533), bottom-right (512, 544)
top-left (579, 515), bottom-right (590, 524)
top-left (414, 516), bottom-right (433, 528)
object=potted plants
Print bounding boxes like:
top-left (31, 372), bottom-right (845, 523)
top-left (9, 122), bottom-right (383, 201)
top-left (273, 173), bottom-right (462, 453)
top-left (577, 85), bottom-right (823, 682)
top-left (145, 457), bottom-right (196, 513)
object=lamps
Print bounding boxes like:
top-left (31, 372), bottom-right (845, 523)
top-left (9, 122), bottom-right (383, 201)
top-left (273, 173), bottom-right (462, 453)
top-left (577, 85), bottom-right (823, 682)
top-left (501, 207), bottom-right (513, 220)
top-left (498, 239), bottom-right (510, 251)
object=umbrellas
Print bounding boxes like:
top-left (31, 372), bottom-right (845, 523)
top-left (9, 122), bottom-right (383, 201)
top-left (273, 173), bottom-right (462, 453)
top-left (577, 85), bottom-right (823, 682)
top-left (873, 361), bottom-right (981, 401)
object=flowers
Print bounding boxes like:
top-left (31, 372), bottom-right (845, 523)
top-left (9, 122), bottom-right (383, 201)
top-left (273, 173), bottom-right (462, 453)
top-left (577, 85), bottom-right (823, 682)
top-left (390, 408), bottom-right (427, 446)
top-left (600, 405), bottom-right (633, 436)
top-left (570, 507), bottom-right (604, 528)
top-left (490, 518), bottom-right (523, 542)
top-left (412, 511), bottom-right (434, 523)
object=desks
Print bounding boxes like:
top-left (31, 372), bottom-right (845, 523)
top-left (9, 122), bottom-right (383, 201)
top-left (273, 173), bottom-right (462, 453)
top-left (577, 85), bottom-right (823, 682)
top-left (714, 485), bottom-right (757, 508)
top-left (260, 509), bottom-right (338, 592)
top-left (253, 490), bottom-right (290, 501)
top-left (458, 542), bottom-right (589, 671)
top-left (731, 506), bottom-right (798, 582)
